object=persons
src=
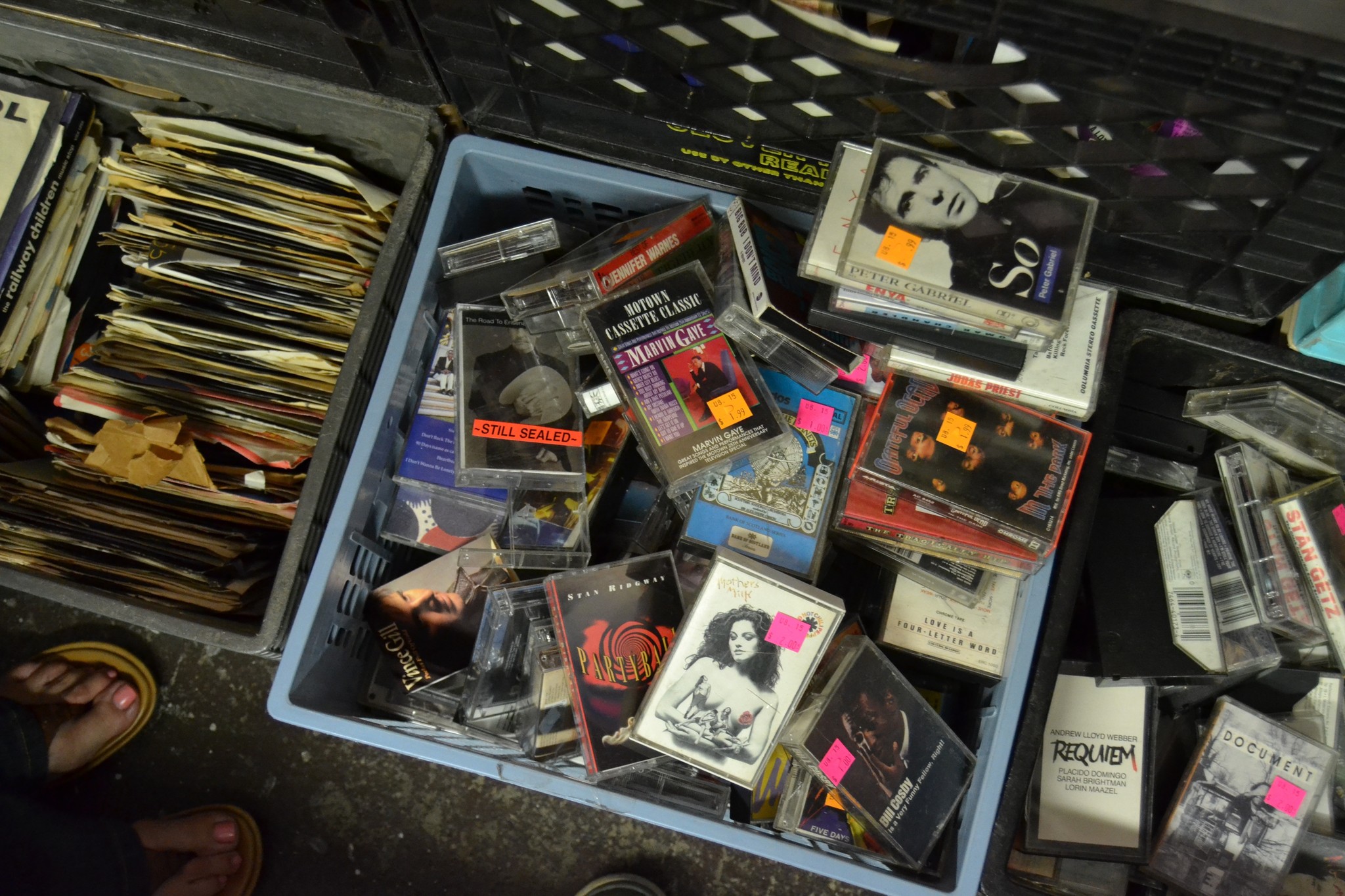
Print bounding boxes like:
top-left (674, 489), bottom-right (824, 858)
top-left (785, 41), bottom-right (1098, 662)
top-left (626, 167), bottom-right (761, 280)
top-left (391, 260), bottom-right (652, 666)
top-left (433, 350), bottom-right (455, 396)
top-left (897, 389), bottom-right (1053, 513)
top-left (858, 142), bottom-right (1088, 322)
top-left (687, 356), bottom-right (728, 422)
top-left (469, 325), bottom-right (577, 473)
top-left (0, 640), bottom-right (264, 896)
top-left (1229, 782), bottom-right (1275, 834)
top-left (654, 604), bottom-right (783, 765)
top-left (366, 560), bottom-right (514, 689)
top-left (837, 665), bottom-right (973, 862)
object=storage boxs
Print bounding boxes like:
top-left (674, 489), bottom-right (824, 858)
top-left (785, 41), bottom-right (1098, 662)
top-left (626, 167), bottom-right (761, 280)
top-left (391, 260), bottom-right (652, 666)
top-left (0, 0), bottom-right (1345, 896)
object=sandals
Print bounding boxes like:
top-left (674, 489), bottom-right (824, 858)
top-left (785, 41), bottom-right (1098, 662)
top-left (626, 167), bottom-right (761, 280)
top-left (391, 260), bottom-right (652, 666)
top-left (0, 805), bottom-right (263, 896)
top-left (0, 640), bottom-right (160, 790)
